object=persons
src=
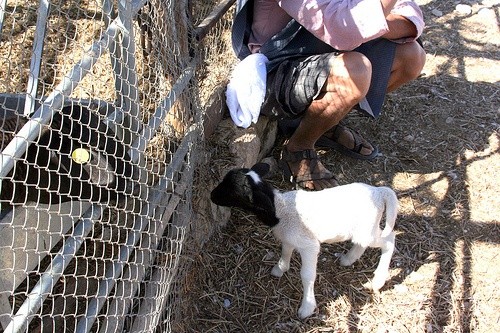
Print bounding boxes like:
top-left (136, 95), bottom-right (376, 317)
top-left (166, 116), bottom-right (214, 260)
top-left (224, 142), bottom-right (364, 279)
top-left (232, 0), bottom-right (426, 190)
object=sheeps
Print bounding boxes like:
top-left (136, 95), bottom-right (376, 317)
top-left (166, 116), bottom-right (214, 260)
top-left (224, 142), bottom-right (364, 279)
top-left (210, 162), bottom-right (399, 318)
top-left (0, 105), bottom-right (134, 221)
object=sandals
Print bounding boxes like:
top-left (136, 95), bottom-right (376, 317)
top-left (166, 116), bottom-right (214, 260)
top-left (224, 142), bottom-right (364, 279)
top-left (316, 120), bottom-right (379, 160)
top-left (275, 142), bottom-right (339, 190)
